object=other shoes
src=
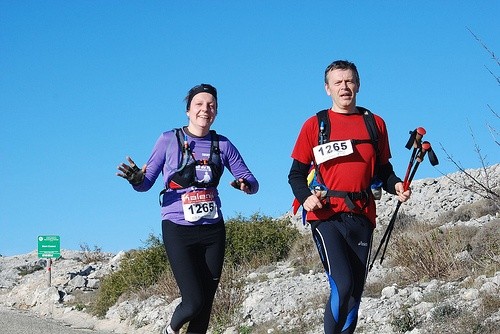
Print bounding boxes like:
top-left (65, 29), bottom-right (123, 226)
top-left (162, 324), bottom-right (178, 334)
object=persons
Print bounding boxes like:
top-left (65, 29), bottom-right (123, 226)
top-left (116, 84), bottom-right (259, 334)
top-left (287, 60), bottom-right (411, 334)
